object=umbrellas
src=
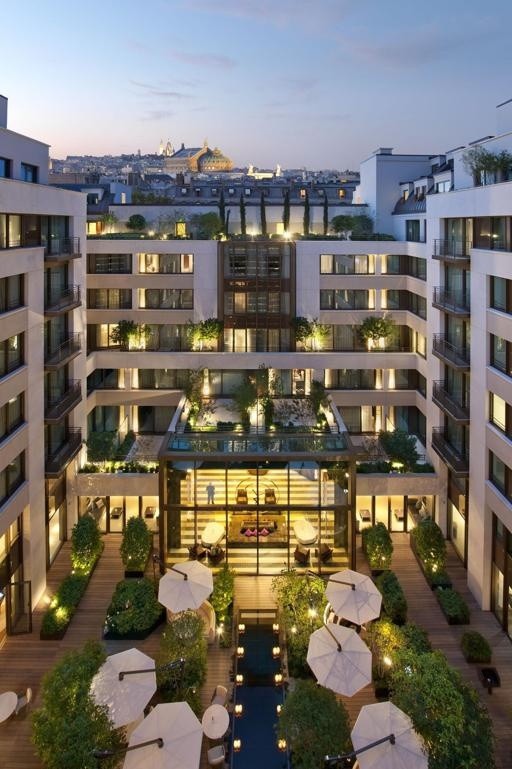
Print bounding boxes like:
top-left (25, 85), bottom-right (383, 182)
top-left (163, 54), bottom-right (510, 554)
top-left (302, 565), bottom-right (384, 628)
top-left (201, 704), bottom-right (231, 742)
top-left (94, 697), bottom-right (203, 769)
top-left (322, 700), bottom-right (431, 769)
top-left (151, 550), bottom-right (214, 615)
top-left (306, 613), bottom-right (374, 699)
top-left (88, 646), bottom-right (187, 734)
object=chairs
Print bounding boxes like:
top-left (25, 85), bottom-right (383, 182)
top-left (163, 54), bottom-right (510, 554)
top-left (210, 685), bottom-right (228, 706)
top-left (188, 543), bottom-right (206, 561)
top-left (207, 745), bottom-right (225, 765)
top-left (207, 544), bottom-right (225, 565)
top-left (16, 688), bottom-right (32, 715)
top-left (264, 489), bottom-right (276, 504)
top-left (294, 543), bottom-right (310, 563)
top-left (314, 543), bottom-right (333, 564)
top-left (237, 489), bottom-right (248, 504)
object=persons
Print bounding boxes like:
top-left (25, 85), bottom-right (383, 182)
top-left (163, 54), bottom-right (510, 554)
top-left (418, 496), bottom-right (429, 516)
top-left (204, 482), bottom-right (215, 505)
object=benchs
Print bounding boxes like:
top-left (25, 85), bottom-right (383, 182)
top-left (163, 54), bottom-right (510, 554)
top-left (295, 519), bottom-right (317, 545)
top-left (201, 523), bottom-right (226, 547)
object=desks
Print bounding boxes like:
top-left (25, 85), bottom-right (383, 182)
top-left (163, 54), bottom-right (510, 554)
top-left (0, 691), bottom-right (17, 723)
